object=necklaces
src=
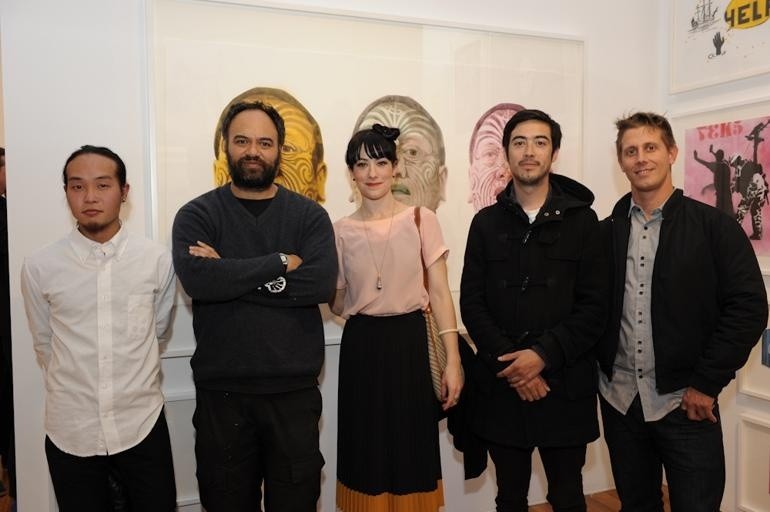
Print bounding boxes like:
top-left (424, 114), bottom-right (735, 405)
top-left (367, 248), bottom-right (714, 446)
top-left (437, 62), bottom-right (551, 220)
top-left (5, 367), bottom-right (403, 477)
top-left (361, 201), bottom-right (394, 290)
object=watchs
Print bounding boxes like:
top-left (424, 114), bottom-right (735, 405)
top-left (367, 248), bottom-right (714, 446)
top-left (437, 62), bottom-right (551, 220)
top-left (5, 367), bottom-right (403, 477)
top-left (279, 252), bottom-right (288, 273)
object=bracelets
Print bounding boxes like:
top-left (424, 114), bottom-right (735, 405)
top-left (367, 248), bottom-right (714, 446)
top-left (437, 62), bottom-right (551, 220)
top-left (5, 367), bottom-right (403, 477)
top-left (439, 329), bottom-right (458, 337)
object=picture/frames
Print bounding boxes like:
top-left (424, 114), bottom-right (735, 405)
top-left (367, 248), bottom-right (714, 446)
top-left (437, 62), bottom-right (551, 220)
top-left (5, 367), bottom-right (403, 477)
top-left (667, 0), bottom-right (770, 93)
top-left (735, 413), bottom-right (770, 511)
top-left (668, 96), bottom-right (770, 274)
top-left (736, 271), bottom-right (769, 401)
top-left (144, 2), bottom-right (588, 360)
top-left (161, 394), bottom-right (199, 509)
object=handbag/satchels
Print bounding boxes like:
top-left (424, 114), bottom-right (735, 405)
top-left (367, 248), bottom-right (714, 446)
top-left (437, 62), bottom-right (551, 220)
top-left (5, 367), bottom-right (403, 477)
top-left (426, 313), bottom-right (465, 401)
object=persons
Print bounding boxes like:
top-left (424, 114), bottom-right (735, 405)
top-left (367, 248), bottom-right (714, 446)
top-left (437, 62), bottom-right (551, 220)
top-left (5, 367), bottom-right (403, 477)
top-left (172, 101), bottom-right (337, 511)
top-left (596, 114), bottom-right (768, 511)
top-left (329, 124), bottom-right (463, 512)
top-left (212, 87), bottom-right (449, 217)
top-left (21, 146), bottom-right (180, 512)
top-left (458, 110), bottom-right (609, 512)
